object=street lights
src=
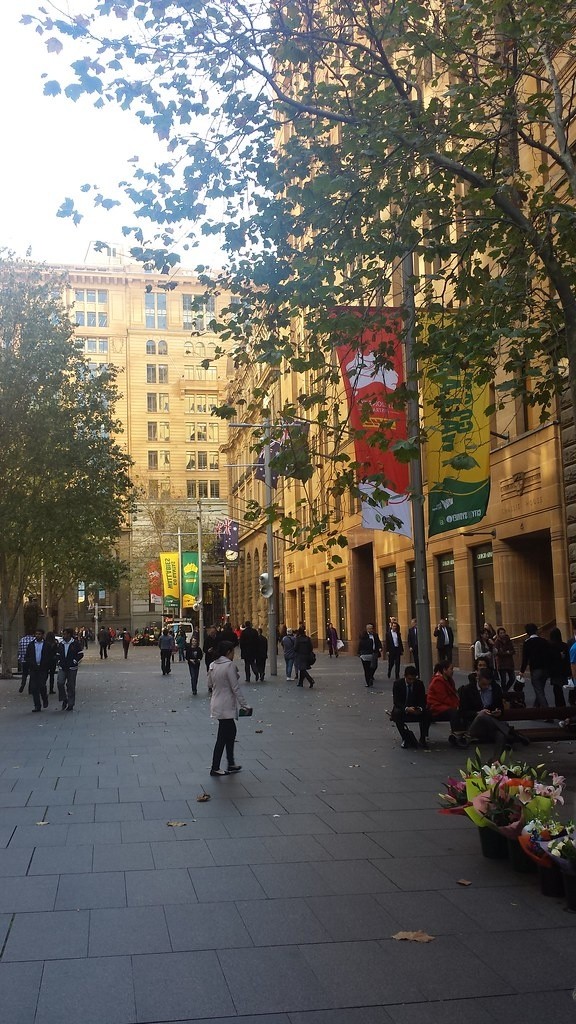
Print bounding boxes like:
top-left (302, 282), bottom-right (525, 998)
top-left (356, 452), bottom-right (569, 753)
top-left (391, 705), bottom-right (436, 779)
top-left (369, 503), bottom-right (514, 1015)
top-left (176, 509), bottom-right (222, 654)
top-left (162, 526), bottom-right (194, 625)
top-left (227, 422), bottom-right (302, 676)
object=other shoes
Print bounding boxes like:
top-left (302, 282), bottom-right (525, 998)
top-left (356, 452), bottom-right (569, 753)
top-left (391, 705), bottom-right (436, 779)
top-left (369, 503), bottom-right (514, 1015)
top-left (228, 765), bottom-right (242, 771)
top-left (449, 735), bottom-right (470, 749)
top-left (210, 768), bottom-right (229, 776)
top-left (32, 707), bottom-right (42, 712)
top-left (245, 679), bottom-right (250, 682)
top-left (297, 684), bottom-right (304, 687)
top-left (401, 739), bottom-right (404, 748)
top-left (287, 677), bottom-right (295, 681)
top-left (543, 717), bottom-right (554, 723)
top-left (43, 700), bottom-right (48, 708)
top-left (256, 675), bottom-right (264, 682)
top-left (309, 680), bottom-right (315, 688)
top-left (418, 737), bottom-right (429, 750)
top-left (365, 678), bottom-right (373, 687)
top-left (61, 699), bottom-right (73, 711)
top-left (19, 684), bottom-right (25, 693)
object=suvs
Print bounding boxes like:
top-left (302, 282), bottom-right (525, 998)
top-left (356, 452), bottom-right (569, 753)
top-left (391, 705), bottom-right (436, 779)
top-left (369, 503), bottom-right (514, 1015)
top-left (168, 623), bottom-right (194, 648)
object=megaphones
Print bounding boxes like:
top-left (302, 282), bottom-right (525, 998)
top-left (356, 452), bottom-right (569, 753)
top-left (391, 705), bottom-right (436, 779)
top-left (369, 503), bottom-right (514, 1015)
top-left (193, 603), bottom-right (200, 611)
top-left (260, 572), bottom-right (269, 585)
top-left (194, 596), bottom-right (202, 603)
top-left (259, 585), bottom-right (273, 599)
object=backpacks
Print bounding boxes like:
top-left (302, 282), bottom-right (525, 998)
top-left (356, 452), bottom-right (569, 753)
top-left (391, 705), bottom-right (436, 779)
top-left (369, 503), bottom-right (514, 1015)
top-left (124, 632), bottom-right (131, 642)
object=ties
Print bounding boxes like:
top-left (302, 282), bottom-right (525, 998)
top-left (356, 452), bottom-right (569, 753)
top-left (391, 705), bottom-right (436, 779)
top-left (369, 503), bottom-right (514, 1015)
top-left (444, 628), bottom-right (447, 639)
top-left (407, 684), bottom-right (412, 707)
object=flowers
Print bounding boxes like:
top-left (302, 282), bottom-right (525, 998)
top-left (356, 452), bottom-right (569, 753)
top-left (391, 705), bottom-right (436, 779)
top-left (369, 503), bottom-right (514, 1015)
top-left (434, 744), bottom-right (576, 872)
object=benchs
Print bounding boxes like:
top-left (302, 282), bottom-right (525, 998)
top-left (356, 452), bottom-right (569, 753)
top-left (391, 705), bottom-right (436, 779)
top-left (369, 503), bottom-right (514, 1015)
top-left (471, 706), bottom-right (576, 745)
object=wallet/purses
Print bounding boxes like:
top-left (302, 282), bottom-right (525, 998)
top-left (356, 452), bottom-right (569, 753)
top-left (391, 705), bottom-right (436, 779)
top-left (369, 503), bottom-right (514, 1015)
top-left (239, 707), bottom-right (252, 717)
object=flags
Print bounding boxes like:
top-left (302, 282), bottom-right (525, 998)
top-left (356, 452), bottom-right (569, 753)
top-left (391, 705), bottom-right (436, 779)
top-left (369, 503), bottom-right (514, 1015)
top-left (254, 437), bottom-right (285, 490)
top-left (213, 519), bottom-right (239, 562)
top-left (280, 416), bottom-right (310, 480)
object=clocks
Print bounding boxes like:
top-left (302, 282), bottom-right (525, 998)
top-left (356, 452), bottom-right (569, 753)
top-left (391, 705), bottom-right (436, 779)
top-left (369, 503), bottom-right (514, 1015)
top-left (225, 549), bottom-right (239, 561)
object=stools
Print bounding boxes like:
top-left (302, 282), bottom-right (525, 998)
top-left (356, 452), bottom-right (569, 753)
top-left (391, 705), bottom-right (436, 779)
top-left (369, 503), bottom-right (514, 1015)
top-left (385, 709), bottom-right (455, 750)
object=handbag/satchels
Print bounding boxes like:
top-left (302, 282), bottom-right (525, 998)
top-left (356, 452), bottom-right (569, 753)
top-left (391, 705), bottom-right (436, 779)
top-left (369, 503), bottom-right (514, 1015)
top-left (513, 675), bottom-right (525, 692)
top-left (310, 652), bottom-right (315, 664)
top-left (401, 723), bottom-right (419, 748)
top-left (337, 640), bottom-right (344, 650)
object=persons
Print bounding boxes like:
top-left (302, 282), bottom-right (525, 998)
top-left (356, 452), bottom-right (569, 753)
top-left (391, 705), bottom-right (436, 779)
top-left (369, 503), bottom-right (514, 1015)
top-left (18, 617), bottom-right (576, 774)
top-left (208, 642), bottom-right (252, 777)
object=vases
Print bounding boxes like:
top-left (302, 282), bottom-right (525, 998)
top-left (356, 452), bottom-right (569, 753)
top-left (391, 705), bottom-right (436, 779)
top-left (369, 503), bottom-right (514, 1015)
top-left (507, 838), bottom-right (538, 874)
top-left (561, 868), bottom-right (576, 914)
top-left (478, 826), bottom-right (510, 859)
top-left (538, 860), bottom-right (566, 897)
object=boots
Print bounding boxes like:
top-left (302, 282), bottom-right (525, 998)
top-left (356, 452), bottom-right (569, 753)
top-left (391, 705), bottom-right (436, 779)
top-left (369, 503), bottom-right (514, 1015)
top-left (509, 726), bottom-right (531, 746)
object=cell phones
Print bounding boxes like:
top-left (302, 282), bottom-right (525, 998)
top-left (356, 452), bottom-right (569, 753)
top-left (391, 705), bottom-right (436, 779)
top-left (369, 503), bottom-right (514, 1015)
top-left (239, 708), bottom-right (252, 716)
